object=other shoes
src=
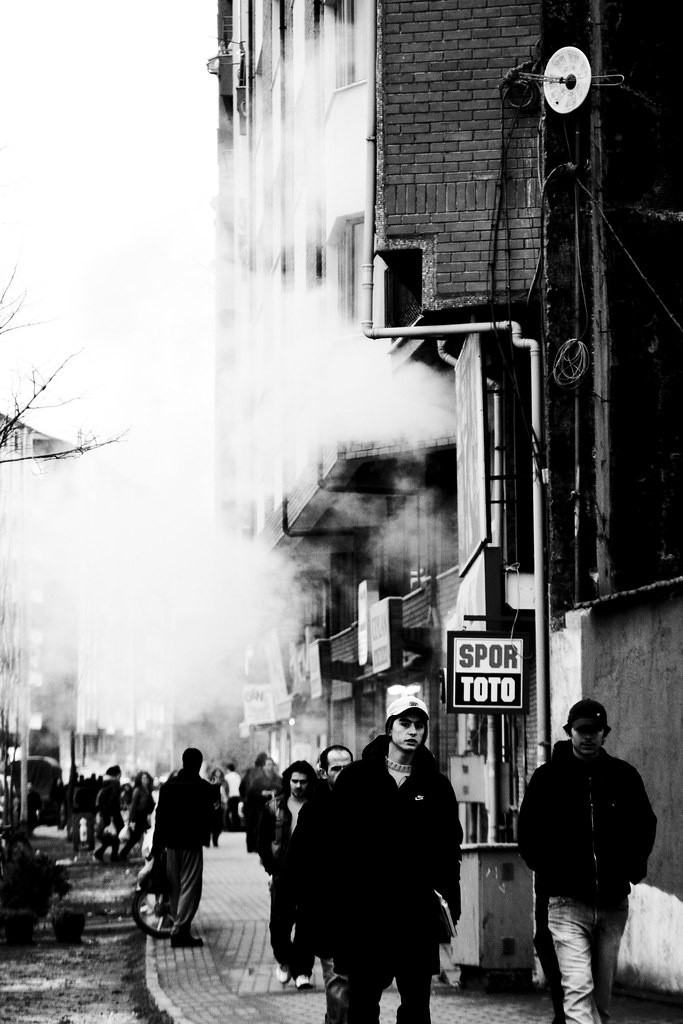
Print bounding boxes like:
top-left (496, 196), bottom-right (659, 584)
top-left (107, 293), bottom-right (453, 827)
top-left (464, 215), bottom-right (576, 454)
top-left (296, 975), bottom-right (310, 989)
top-left (170, 933), bottom-right (203, 949)
top-left (119, 850), bottom-right (132, 867)
top-left (94, 850), bottom-right (109, 864)
top-left (276, 962), bottom-right (291, 984)
top-left (110, 852), bottom-right (120, 861)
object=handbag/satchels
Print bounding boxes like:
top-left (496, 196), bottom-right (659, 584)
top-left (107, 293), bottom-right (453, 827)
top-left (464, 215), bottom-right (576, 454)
top-left (119, 823), bottom-right (130, 843)
top-left (104, 818), bottom-right (116, 837)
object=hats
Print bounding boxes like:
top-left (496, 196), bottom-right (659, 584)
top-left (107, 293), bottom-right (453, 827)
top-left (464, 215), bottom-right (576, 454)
top-left (567, 698), bottom-right (607, 730)
top-left (385, 696), bottom-right (430, 725)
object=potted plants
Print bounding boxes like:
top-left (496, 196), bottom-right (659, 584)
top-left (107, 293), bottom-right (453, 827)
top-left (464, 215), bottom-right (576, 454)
top-left (52, 901), bottom-right (85, 945)
top-left (0, 846), bottom-right (73, 948)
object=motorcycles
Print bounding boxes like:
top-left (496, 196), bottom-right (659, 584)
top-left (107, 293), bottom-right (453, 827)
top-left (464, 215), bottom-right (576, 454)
top-left (131, 849), bottom-right (174, 939)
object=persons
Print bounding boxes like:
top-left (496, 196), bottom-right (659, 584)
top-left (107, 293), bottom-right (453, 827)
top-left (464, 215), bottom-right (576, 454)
top-left (317, 695), bottom-right (464, 1023)
top-left (256, 760), bottom-right (317, 990)
top-left (0, 748), bottom-right (353, 990)
top-left (119, 770), bottom-right (156, 864)
top-left (145, 748), bottom-right (216, 951)
top-left (516, 699), bottom-right (659, 1024)
top-left (94, 764), bottom-right (124, 863)
